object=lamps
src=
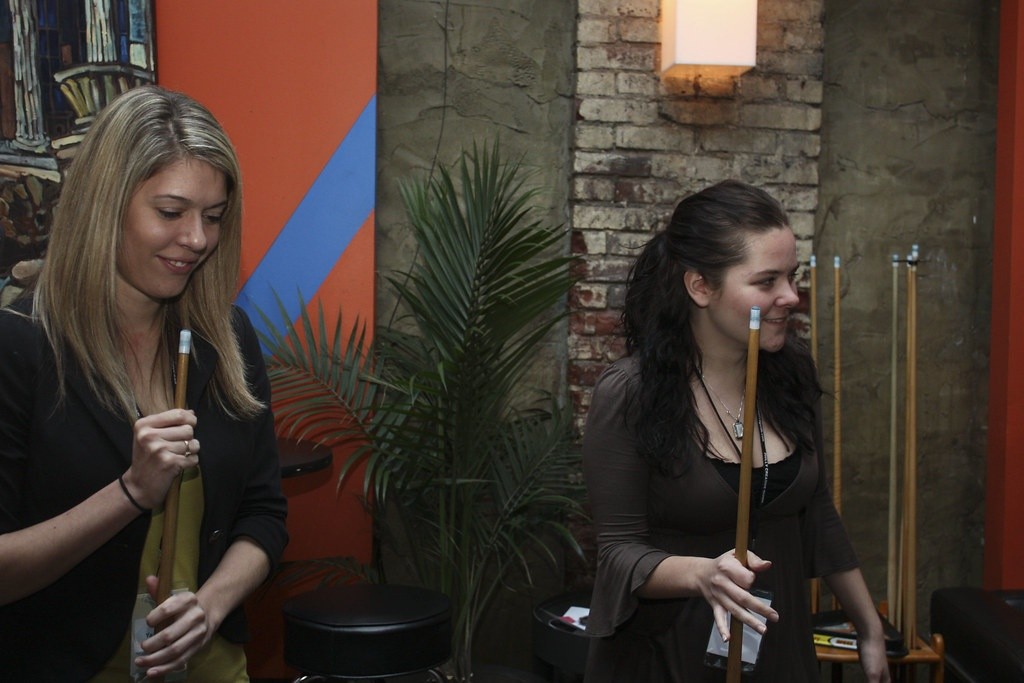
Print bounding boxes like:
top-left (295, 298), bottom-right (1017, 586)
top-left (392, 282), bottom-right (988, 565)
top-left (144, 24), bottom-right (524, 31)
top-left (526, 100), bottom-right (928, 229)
top-left (658, 0), bottom-right (756, 77)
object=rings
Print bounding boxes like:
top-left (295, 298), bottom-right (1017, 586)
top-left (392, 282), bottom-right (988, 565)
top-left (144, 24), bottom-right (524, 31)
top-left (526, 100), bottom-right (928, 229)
top-left (184, 440), bottom-right (192, 458)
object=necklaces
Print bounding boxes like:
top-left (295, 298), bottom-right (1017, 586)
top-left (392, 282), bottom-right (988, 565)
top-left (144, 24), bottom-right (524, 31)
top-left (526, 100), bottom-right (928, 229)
top-left (702, 374), bottom-right (745, 439)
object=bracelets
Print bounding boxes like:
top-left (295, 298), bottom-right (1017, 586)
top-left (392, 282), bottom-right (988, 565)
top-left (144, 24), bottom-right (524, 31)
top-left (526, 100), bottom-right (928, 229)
top-left (118, 474), bottom-right (146, 511)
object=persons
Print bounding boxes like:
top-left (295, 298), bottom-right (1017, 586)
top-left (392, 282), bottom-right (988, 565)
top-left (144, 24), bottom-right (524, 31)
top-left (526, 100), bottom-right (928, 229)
top-left (584, 179), bottom-right (891, 683)
top-left (1, 84), bottom-right (291, 683)
